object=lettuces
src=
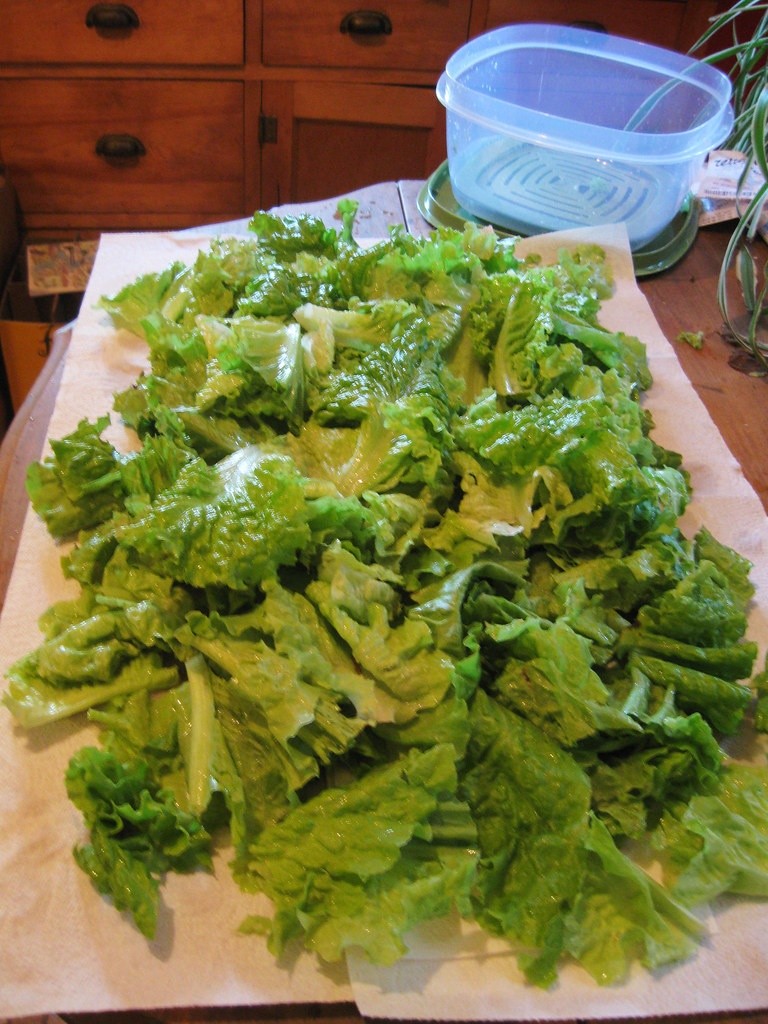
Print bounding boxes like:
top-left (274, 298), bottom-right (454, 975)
top-left (2, 195), bottom-right (768, 991)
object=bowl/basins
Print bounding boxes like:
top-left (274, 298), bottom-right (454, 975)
top-left (434, 23), bottom-right (736, 253)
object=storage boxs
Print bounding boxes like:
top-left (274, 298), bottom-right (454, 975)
top-left (411, 20), bottom-right (738, 278)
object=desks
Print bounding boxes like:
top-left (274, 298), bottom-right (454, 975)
top-left (0, 182), bottom-right (768, 1024)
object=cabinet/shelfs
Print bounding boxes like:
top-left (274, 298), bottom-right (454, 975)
top-left (1, 1), bottom-right (767, 316)
